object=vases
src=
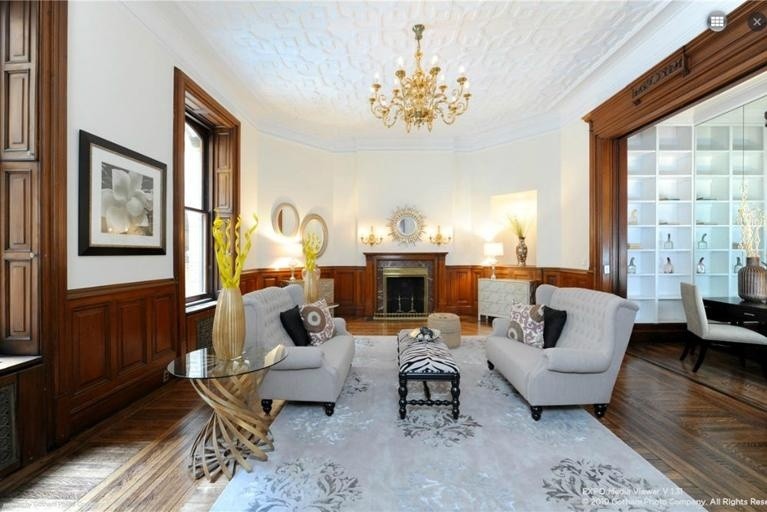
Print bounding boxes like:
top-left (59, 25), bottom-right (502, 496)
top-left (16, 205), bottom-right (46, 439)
top-left (515, 237), bottom-right (528, 266)
top-left (300, 267), bottom-right (320, 306)
top-left (210, 286), bottom-right (247, 361)
top-left (736, 254), bottom-right (766, 306)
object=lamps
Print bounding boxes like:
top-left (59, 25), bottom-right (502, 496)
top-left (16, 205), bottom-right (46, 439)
top-left (358, 224), bottom-right (386, 246)
top-left (428, 224), bottom-right (452, 247)
top-left (366, 23), bottom-right (473, 135)
top-left (482, 241), bottom-right (505, 280)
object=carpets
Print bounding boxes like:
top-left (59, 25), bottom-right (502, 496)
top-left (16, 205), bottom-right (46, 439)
top-left (197, 332), bottom-right (713, 512)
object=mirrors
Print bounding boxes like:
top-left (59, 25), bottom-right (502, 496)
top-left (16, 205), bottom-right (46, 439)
top-left (271, 202), bottom-right (300, 241)
top-left (300, 213), bottom-right (328, 260)
top-left (384, 204), bottom-right (428, 249)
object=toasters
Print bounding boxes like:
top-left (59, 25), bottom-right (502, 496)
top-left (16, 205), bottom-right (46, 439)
top-left (697, 256), bottom-right (705, 274)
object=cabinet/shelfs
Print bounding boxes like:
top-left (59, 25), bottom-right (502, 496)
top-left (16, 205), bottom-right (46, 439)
top-left (624, 120), bottom-right (766, 325)
top-left (476, 276), bottom-right (541, 323)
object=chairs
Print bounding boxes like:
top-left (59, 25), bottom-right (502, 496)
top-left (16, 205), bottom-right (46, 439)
top-left (676, 281), bottom-right (767, 380)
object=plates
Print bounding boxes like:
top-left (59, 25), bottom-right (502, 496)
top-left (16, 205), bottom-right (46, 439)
top-left (409, 327), bottom-right (442, 342)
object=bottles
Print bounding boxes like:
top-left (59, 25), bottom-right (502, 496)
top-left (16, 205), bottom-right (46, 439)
top-left (628, 207), bottom-right (638, 225)
top-left (628, 257), bottom-right (636, 273)
top-left (697, 233), bottom-right (709, 248)
top-left (663, 257), bottom-right (674, 274)
top-left (734, 257), bottom-right (743, 272)
top-left (663, 233), bottom-right (673, 248)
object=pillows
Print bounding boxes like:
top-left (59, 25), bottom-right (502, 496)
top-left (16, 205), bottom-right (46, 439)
top-left (541, 304), bottom-right (567, 350)
top-left (503, 297), bottom-right (546, 351)
top-left (298, 298), bottom-right (337, 347)
top-left (279, 304), bottom-right (312, 348)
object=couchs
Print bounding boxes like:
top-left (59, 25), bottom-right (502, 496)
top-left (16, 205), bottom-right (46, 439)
top-left (238, 284), bottom-right (355, 417)
top-left (484, 283), bottom-right (639, 420)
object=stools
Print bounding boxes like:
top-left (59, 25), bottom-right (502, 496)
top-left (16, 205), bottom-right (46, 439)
top-left (425, 312), bottom-right (463, 350)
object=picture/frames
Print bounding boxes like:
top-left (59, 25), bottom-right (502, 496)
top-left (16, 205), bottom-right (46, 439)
top-left (77, 128), bottom-right (167, 258)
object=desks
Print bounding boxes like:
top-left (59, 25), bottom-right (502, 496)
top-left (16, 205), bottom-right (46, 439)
top-left (164, 341), bottom-right (289, 483)
top-left (326, 302), bottom-right (340, 318)
top-left (700, 294), bottom-right (766, 380)
